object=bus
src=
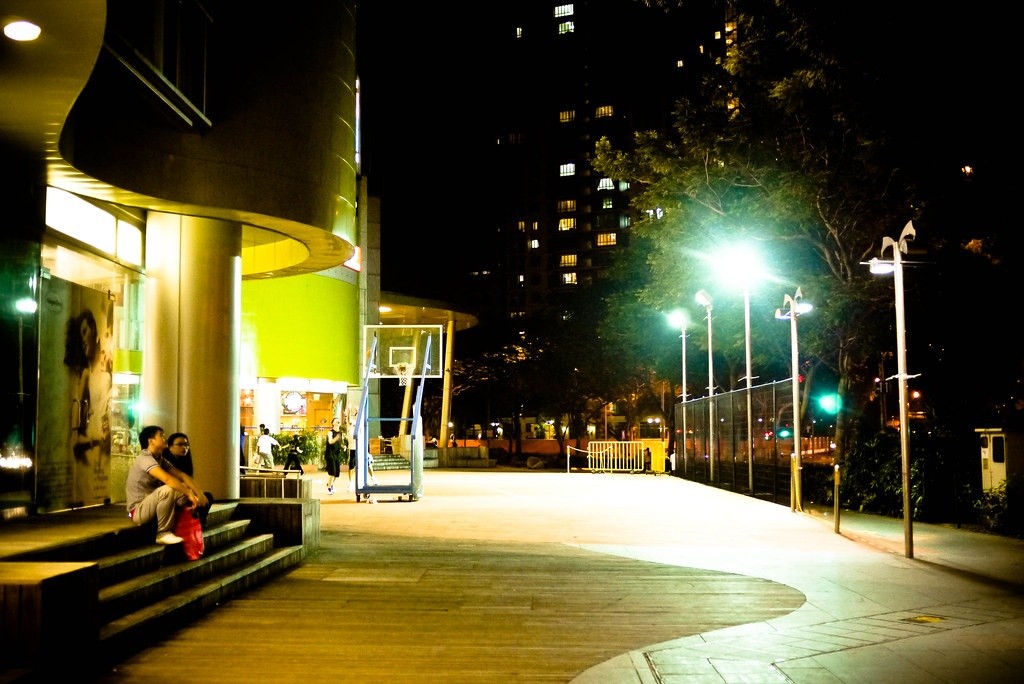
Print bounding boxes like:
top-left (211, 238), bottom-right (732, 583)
top-left (671, 373), bottom-right (855, 467)
top-left (425, 409), bottom-right (670, 463)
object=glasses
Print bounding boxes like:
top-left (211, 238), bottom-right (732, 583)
top-left (173, 442), bottom-right (190, 447)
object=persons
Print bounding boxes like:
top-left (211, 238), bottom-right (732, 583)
top-left (257, 424), bottom-right (304, 475)
top-left (126, 426), bottom-right (214, 543)
top-left (348, 408), bottom-right (378, 492)
top-left (64, 309), bottom-right (112, 503)
top-left (324, 418), bottom-right (342, 495)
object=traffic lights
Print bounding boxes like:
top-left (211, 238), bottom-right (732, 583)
top-left (819, 392), bottom-right (836, 410)
top-left (778, 430), bottom-right (789, 437)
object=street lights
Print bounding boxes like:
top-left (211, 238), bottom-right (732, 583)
top-left (646, 416), bottom-right (665, 441)
top-left (695, 289), bottom-right (716, 482)
top-left (17, 296), bottom-right (39, 419)
top-left (857, 241), bottom-right (917, 559)
top-left (712, 240), bottom-right (769, 494)
top-left (669, 314), bottom-right (692, 479)
top-left (774, 287), bottom-right (813, 513)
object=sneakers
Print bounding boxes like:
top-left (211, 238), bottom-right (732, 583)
top-left (156, 531), bottom-right (184, 545)
top-left (327, 483), bottom-right (335, 495)
